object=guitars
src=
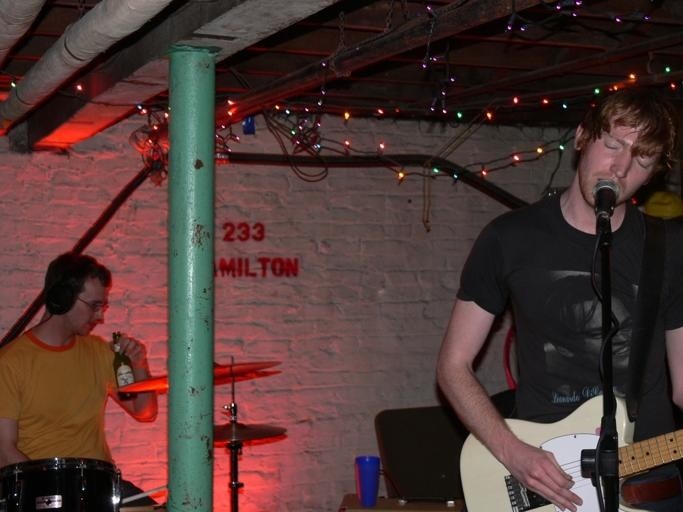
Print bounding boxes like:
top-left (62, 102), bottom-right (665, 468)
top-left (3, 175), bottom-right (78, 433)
top-left (460, 393), bottom-right (683, 512)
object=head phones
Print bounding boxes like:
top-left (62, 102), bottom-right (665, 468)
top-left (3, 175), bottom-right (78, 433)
top-left (44, 256), bottom-right (96, 316)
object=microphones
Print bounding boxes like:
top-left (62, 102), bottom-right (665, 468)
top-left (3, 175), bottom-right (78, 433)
top-left (592, 178), bottom-right (621, 251)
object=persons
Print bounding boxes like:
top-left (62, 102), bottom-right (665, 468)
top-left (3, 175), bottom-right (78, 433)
top-left (437, 89), bottom-right (682, 512)
top-left (0, 251), bottom-right (162, 510)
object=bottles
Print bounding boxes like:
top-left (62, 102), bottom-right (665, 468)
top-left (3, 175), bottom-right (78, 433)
top-left (112, 331), bottom-right (137, 400)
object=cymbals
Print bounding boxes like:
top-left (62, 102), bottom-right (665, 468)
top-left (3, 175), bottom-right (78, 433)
top-left (116, 362), bottom-right (283, 394)
top-left (214, 418), bottom-right (287, 448)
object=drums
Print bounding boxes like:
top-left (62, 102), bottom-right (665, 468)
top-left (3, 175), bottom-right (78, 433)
top-left (2, 456), bottom-right (122, 512)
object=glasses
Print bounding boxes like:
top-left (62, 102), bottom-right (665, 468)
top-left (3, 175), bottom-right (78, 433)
top-left (78, 297), bottom-right (110, 313)
